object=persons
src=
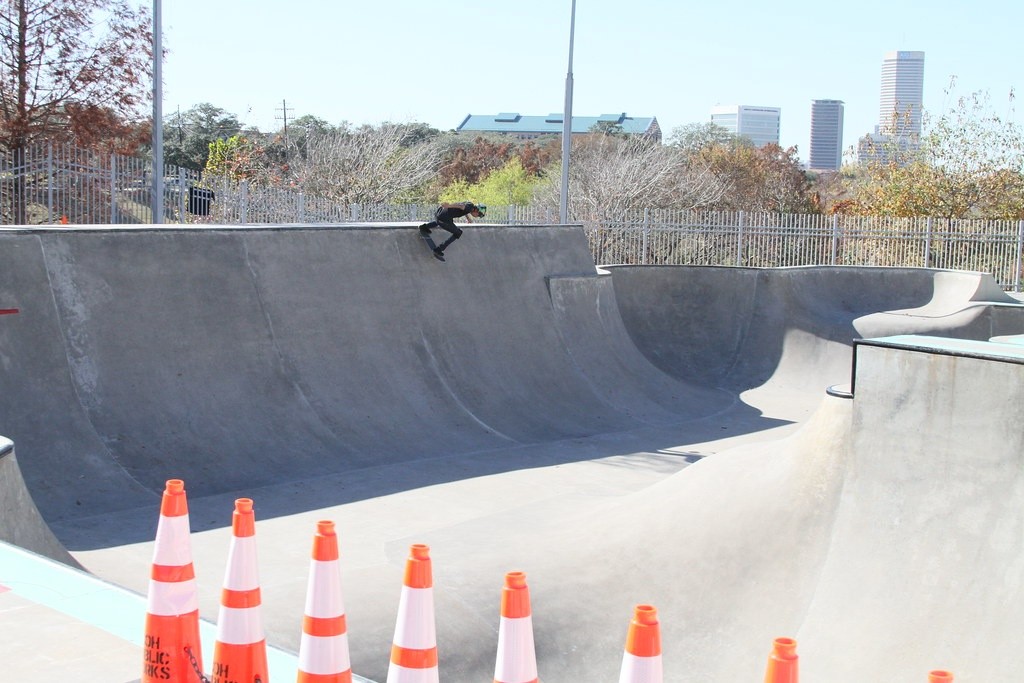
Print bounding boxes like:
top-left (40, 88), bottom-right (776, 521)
top-left (417, 201), bottom-right (487, 258)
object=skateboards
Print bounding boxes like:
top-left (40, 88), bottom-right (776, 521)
top-left (418, 223), bottom-right (446, 262)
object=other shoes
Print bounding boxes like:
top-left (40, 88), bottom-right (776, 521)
top-left (433, 247), bottom-right (444, 256)
top-left (420, 224), bottom-right (432, 234)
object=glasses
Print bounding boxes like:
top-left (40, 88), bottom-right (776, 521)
top-left (476, 210), bottom-right (483, 218)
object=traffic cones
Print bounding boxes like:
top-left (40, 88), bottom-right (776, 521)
top-left (761, 635), bottom-right (799, 683)
top-left (927, 670), bottom-right (955, 682)
top-left (205, 497), bottom-right (276, 683)
top-left (138, 480), bottom-right (207, 682)
top-left (615, 606), bottom-right (665, 683)
top-left (383, 541), bottom-right (442, 683)
top-left (292, 518), bottom-right (360, 682)
top-left (491, 570), bottom-right (542, 682)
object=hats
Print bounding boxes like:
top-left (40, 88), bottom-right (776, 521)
top-left (475, 203), bottom-right (487, 216)
